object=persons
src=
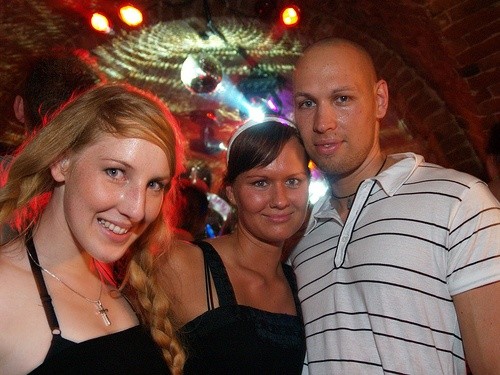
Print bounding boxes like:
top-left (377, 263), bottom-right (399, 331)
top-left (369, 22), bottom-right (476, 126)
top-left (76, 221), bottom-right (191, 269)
top-left (152, 115), bottom-right (311, 375)
top-left (0, 83), bottom-right (187, 375)
top-left (171, 178), bottom-right (208, 243)
top-left (0, 54), bottom-right (107, 245)
top-left (282, 37), bottom-right (500, 375)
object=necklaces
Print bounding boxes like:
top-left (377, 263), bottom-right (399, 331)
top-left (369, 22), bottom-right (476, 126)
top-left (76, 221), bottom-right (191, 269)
top-left (332, 159), bottom-right (386, 209)
top-left (21, 238), bottom-right (112, 326)
